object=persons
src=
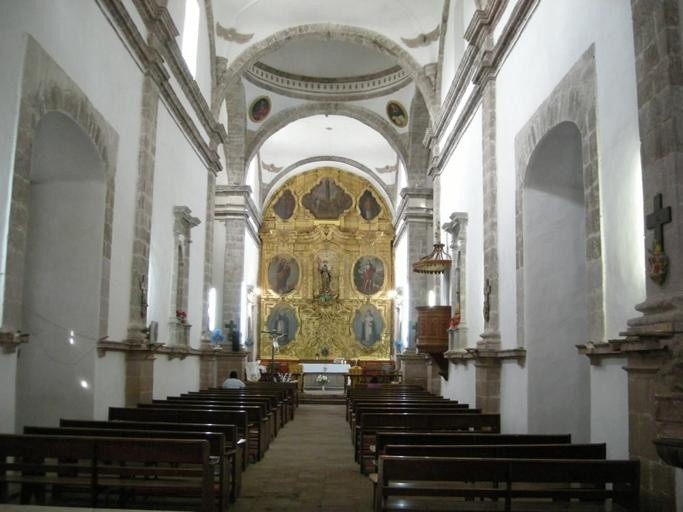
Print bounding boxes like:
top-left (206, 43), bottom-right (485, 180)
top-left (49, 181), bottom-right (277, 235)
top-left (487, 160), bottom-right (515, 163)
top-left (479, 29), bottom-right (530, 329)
top-left (367, 376), bottom-right (382, 389)
top-left (222, 371), bottom-right (246, 390)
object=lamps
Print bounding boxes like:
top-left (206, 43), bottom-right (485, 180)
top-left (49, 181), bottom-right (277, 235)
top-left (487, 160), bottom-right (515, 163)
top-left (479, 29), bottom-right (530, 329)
top-left (412, 243), bottom-right (452, 274)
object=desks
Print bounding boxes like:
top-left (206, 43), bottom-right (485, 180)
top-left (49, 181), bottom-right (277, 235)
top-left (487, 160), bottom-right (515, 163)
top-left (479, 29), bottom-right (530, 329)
top-left (260, 373), bottom-right (304, 393)
top-left (342, 373), bottom-right (403, 393)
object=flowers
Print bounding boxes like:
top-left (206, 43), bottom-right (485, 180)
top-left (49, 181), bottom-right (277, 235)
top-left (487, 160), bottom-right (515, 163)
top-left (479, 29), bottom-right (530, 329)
top-left (176, 310), bottom-right (187, 323)
top-left (211, 329), bottom-right (224, 343)
top-left (316, 375), bottom-right (330, 385)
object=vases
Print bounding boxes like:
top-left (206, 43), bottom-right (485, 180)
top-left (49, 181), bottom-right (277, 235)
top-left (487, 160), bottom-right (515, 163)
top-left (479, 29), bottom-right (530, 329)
top-left (321, 386), bottom-right (325, 391)
top-left (214, 337), bottom-right (223, 349)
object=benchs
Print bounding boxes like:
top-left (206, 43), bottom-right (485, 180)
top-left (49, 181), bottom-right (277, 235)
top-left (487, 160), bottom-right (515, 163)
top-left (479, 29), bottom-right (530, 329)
top-left (344, 385), bottom-right (641, 512)
top-left (0, 382), bottom-right (298, 512)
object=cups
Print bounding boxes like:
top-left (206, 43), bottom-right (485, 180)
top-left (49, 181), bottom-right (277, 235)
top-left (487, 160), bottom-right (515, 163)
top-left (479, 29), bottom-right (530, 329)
top-left (344, 361), bottom-right (346, 364)
top-left (341, 360), bottom-right (343, 364)
